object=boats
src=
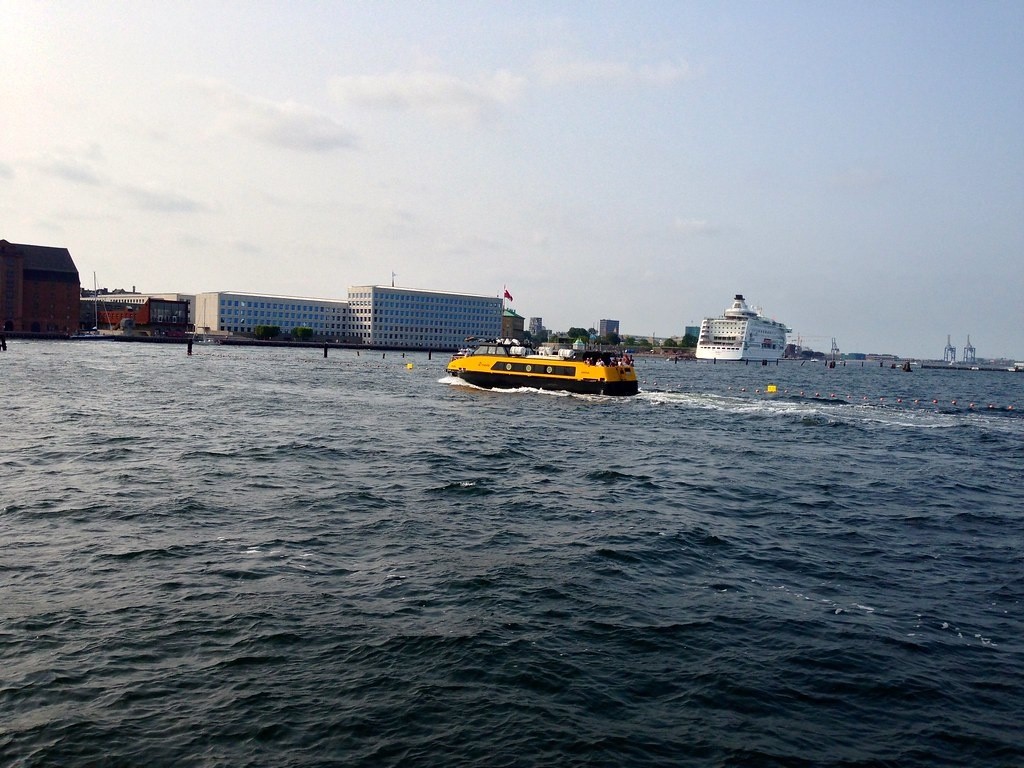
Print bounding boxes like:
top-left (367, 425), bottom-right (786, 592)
top-left (446, 342), bottom-right (638, 396)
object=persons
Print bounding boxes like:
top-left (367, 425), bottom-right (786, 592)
top-left (584, 353), bottom-right (634, 367)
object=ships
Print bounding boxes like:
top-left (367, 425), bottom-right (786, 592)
top-left (696, 294), bottom-right (793, 361)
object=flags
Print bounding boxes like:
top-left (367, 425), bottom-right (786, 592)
top-left (504, 290), bottom-right (513, 301)
